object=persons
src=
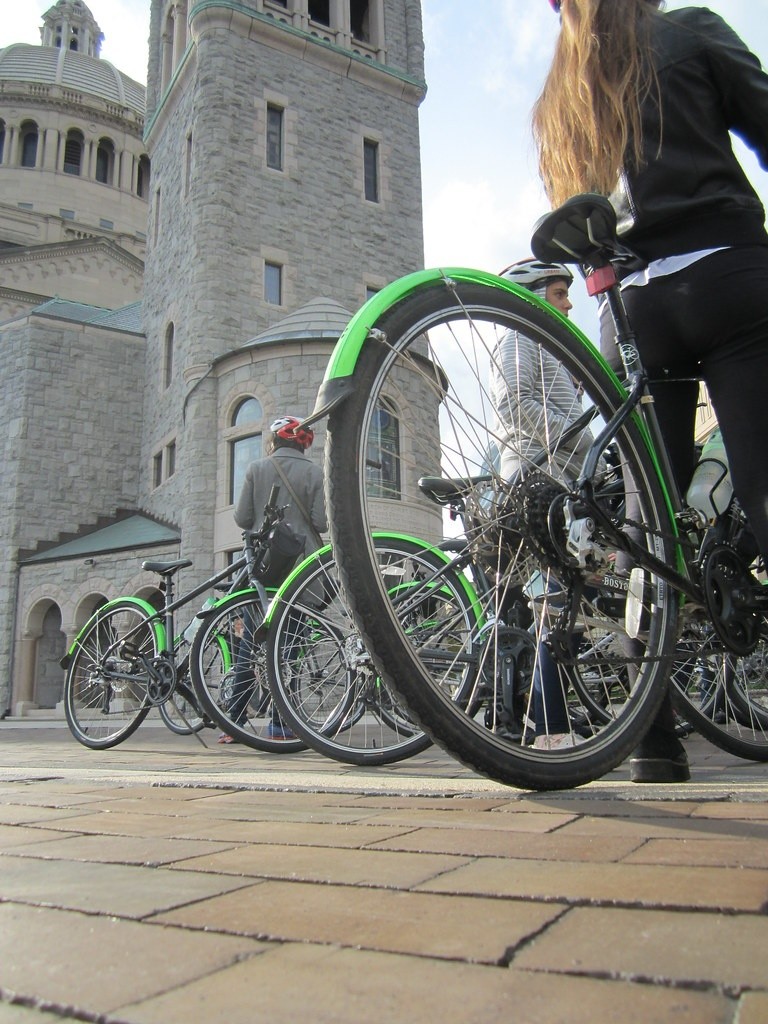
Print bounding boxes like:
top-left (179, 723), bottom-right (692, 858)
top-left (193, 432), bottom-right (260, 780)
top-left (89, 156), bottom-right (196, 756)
top-left (485, 0), bottom-right (768, 781)
top-left (216, 416), bottom-right (329, 742)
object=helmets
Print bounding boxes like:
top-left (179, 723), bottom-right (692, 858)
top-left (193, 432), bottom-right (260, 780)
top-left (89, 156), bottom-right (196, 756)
top-left (271, 416), bottom-right (315, 448)
top-left (499, 258), bottom-right (574, 289)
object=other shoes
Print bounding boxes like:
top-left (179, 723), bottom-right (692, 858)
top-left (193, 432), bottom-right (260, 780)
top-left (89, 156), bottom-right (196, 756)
top-left (533, 732), bottom-right (585, 749)
top-left (630, 733), bottom-right (693, 783)
top-left (499, 720), bottom-right (533, 745)
top-left (709, 708), bottom-right (730, 723)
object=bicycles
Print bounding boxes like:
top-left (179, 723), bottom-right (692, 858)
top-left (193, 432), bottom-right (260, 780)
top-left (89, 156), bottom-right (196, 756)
top-left (57, 192), bottom-right (767, 793)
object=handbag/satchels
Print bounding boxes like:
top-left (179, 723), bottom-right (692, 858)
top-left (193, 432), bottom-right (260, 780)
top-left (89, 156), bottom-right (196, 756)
top-left (316, 577), bottom-right (341, 611)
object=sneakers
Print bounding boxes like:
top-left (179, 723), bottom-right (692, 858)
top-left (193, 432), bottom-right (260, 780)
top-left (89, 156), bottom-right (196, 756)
top-left (218, 730), bottom-right (237, 744)
top-left (269, 721), bottom-right (296, 739)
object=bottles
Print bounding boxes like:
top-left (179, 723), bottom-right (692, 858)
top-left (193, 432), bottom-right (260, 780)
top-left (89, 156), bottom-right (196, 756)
top-left (184, 597), bottom-right (217, 643)
top-left (687, 428), bottom-right (734, 518)
